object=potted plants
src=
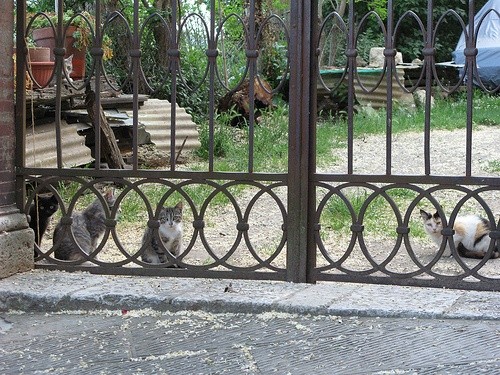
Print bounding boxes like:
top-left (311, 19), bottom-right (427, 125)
top-left (33, 10), bottom-right (114, 81)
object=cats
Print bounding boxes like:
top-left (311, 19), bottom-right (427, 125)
top-left (419, 205), bottom-right (500, 260)
top-left (142, 201), bottom-right (187, 268)
top-left (29, 195), bottom-right (59, 258)
top-left (52, 186), bottom-right (122, 267)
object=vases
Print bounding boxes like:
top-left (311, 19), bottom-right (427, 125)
top-left (28, 62), bottom-right (55, 87)
top-left (13, 47), bottom-right (51, 87)
